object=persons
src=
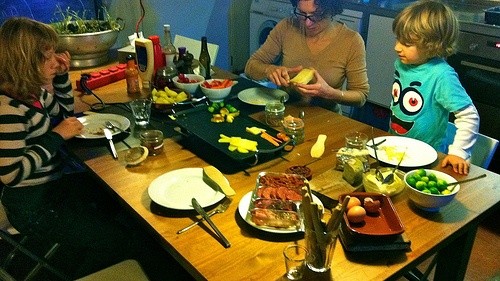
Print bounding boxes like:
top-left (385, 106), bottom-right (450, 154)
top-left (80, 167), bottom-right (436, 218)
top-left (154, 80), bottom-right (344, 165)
top-left (242, 0), bottom-right (370, 116)
top-left (388, 0), bottom-right (480, 175)
top-left (0, 20), bottom-right (129, 279)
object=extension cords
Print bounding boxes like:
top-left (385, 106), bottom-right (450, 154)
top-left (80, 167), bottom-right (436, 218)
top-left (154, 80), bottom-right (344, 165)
top-left (76, 64), bottom-right (127, 92)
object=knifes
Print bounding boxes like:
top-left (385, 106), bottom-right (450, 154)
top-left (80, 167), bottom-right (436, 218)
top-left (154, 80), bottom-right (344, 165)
top-left (192, 197), bottom-right (231, 248)
top-left (102, 126), bottom-right (118, 159)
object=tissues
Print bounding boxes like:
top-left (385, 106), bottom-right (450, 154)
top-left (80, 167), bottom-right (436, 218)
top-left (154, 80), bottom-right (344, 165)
top-left (117, 30), bottom-right (145, 65)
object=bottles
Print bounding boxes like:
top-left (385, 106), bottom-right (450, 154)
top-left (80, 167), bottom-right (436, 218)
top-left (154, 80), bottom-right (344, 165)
top-left (125, 35), bottom-right (163, 93)
top-left (200, 37), bottom-right (210, 79)
top-left (162, 24), bottom-right (176, 67)
top-left (178, 47), bottom-right (186, 62)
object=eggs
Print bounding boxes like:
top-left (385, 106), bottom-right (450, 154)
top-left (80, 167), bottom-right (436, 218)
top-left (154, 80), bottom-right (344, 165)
top-left (345, 196), bottom-right (366, 223)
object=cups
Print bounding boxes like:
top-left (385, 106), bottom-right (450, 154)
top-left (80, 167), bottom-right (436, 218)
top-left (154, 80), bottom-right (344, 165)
top-left (302, 220), bottom-right (338, 275)
top-left (283, 245), bottom-right (309, 280)
top-left (129, 97), bottom-right (152, 126)
top-left (139, 130), bottom-right (164, 156)
top-left (345, 132), bottom-right (368, 150)
top-left (280, 118), bottom-right (304, 144)
top-left (265, 101), bottom-right (286, 127)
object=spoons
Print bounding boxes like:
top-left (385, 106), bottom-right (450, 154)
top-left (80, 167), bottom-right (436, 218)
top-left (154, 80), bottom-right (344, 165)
top-left (382, 152), bottom-right (403, 184)
top-left (371, 137), bottom-right (385, 182)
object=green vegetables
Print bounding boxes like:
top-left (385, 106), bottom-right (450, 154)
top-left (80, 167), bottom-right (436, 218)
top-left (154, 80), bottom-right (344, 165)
top-left (51, 4), bottom-right (121, 34)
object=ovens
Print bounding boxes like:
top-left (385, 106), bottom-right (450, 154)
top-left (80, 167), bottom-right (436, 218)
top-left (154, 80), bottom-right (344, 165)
top-left (446, 30), bottom-right (500, 233)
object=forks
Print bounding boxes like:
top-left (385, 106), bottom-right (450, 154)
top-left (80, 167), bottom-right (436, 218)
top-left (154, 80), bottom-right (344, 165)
top-left (177, 199), bottom-right (233, 233)
top-left (104, 121), bottom-right (134, 135)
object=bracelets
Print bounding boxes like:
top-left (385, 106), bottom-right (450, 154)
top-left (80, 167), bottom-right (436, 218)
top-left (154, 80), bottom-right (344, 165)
top-left (342, 93), bottom-right (344, 96)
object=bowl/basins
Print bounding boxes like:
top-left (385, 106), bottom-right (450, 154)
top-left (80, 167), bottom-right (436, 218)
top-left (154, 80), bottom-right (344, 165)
top-left (199, 79), bottom-right (238, 103)
top-left (339, 192), bottom-right (404, 243)
top-left (172, 73), bottom-right (205, 97)
top-left (403, 169), bottom-right (460, 212)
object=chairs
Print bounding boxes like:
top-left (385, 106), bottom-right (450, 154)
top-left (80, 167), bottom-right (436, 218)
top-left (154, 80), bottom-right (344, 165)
top-left (0, 184), bottom-right (151, 281)
top-left (174, 35), bottom-right (500, 281)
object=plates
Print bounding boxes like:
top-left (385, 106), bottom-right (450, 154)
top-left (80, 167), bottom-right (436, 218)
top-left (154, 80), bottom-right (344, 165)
top-left (149, 89), bottom-right (195, 106)
top-left (238, 87), bottom-right (289, 106)
top-left (147, 167), bottom-right (229, 210)
top-left (366, 136), bottom-right (437, 167)
top-left (238, 190), bottom-right (324, 233)
top-left (73, 114), bottom-right (130, 138)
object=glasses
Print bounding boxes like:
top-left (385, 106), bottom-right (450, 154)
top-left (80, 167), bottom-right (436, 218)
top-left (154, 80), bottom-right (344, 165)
top-left (293, 7), bottom-right (325, 21)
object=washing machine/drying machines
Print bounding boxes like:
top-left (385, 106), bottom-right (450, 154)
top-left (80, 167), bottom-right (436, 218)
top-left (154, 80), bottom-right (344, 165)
top-left (249, 0), bottom-right (297, 59)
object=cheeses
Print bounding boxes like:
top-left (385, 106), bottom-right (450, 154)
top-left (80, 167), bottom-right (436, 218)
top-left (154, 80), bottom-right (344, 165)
top-left (289, 68), bottom-right (314, 84)
top-left (218, 134), bottom-right (258, 153)
top-left (203, 165), bottom-right (236, 196)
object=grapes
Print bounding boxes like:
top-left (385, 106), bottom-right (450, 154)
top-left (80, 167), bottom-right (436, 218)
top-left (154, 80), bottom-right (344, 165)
top-left (177, 73), bottom-right (189, 83)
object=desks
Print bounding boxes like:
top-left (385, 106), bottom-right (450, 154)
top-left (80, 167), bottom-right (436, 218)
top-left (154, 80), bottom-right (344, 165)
top-left (45, 46), bottom-right (500, 281)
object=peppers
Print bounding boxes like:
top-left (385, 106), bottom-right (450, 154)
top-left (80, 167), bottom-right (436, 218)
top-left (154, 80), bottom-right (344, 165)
top-left (202, 78), bottom-right (233, 88)
